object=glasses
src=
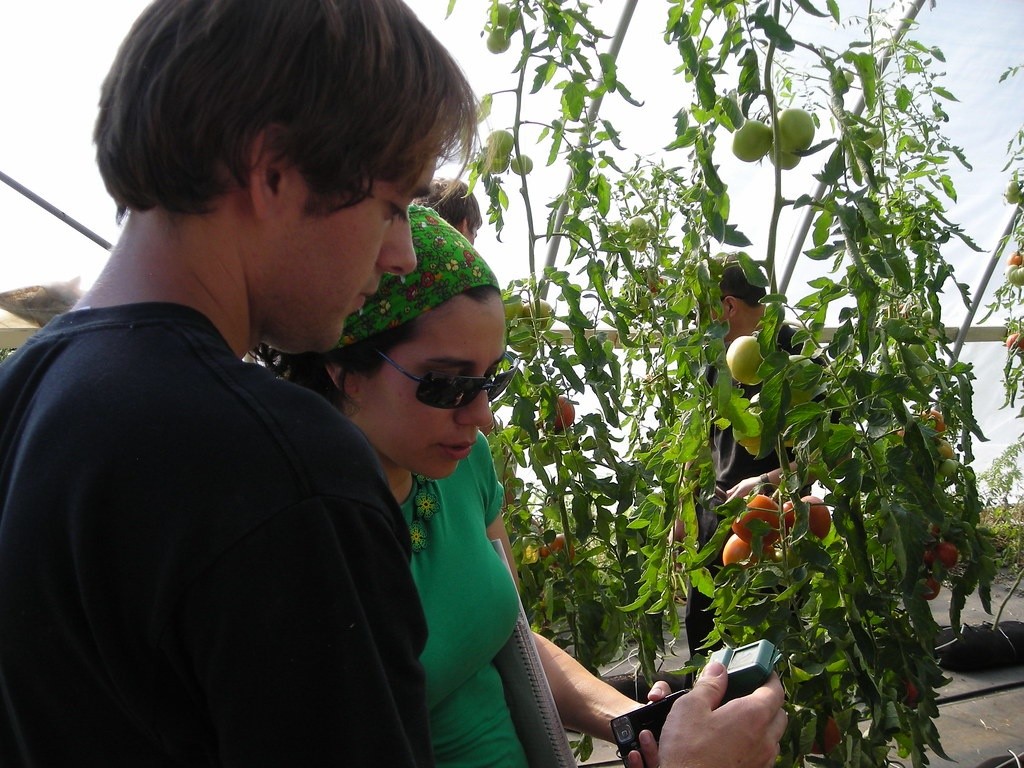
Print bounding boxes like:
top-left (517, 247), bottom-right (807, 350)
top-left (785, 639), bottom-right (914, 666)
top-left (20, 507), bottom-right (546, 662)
top-left (378, 348), bottom-right (523, 410)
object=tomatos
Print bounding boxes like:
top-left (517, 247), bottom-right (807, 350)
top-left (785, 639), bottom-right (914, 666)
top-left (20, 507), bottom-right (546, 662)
top-left (474, 3), bottom-right (1024, 755)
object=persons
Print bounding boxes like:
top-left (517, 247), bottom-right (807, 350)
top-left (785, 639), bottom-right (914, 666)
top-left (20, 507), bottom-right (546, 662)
top-left (410, 176), bottom-right (482, 244)
top-left (245, 201), bottom-right (676, 768)
top-left (685, 256), bottom-right (844, 661)
top-left (0, 0), bottom-right (788, 768)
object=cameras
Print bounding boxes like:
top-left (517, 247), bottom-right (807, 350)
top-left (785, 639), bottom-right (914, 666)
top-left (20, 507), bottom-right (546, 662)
top-left (611, 688), bottom-right (693, 768)
